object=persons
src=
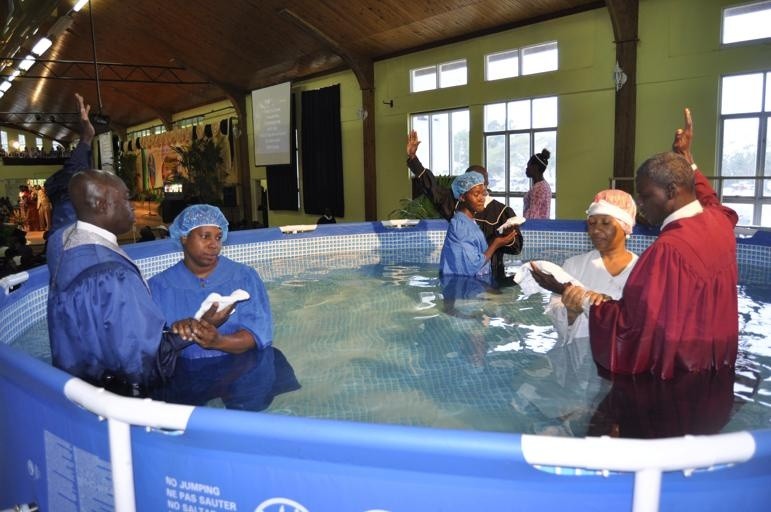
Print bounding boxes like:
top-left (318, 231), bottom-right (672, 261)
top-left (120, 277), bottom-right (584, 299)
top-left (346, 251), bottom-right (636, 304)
top-left (527, 107), bottom-right (740, 438)
top-left (0, 184), bottom-right (52, 279)
top-left (137, 226), bottom-right (156, 242)
top-left (42, 91), bottom-right (237, 397)
top-left (404, 128), bottom-right (525, 290)
top-left (435, 170), bottom-right (519, 289)
top-left (316, 207), bottom-right (336, 224)
top-left (508, 187), bottom-right (641, 432)
top-left (523, 149), bottom-right (554, 219)
top-left (0, 143), bottom-right (76, 161)
top-left (153, 223), bottom-right (170, 239)
top-left (146, 202), bottom-right (303, 412)
